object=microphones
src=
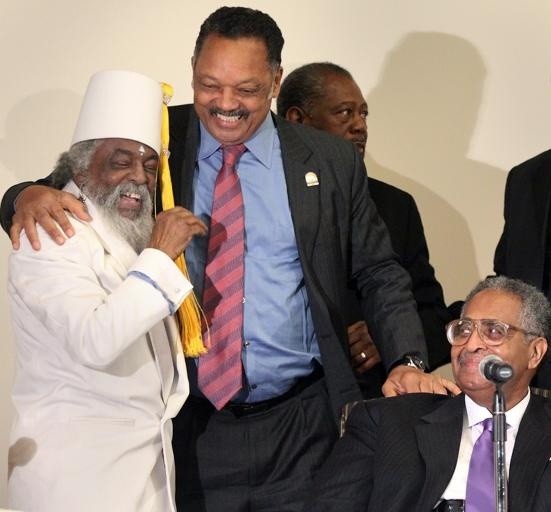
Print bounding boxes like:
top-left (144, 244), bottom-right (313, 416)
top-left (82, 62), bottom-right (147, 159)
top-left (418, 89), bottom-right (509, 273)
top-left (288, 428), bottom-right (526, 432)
top-left (479, 354), bottom-right (515, 384)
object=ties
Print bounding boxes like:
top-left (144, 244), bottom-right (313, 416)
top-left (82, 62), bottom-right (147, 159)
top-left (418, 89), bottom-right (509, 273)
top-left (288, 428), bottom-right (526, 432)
top-left (193, 141), bottom-right (246, 413)
top-left (461, 417), bottom-right (508, 512)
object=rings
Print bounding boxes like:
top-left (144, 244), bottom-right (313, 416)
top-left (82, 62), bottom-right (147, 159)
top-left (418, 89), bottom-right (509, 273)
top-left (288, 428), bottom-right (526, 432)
top-left (359, 350), bottom-right (369, 361)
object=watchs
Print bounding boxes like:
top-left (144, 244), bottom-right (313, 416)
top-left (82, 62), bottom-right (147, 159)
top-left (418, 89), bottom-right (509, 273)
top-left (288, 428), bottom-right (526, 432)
top-left (392, 353), bottom-right (430, 375)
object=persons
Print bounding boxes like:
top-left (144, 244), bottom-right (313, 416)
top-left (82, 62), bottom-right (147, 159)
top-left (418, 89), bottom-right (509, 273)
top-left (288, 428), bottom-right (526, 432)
top-left (443, 147), bottom-right (549, 394)
top-left (273, 60), bottom-right (457, 401)
top-left (0, 5), bottom-right (466, 510)
top-left (0, 137), bottom-right (211, 511)
top-left (300, 276), bottom-right (551, 511)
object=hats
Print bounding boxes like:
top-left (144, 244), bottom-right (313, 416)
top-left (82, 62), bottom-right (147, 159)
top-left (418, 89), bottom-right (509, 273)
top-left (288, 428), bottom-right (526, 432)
top-left (69, 67), bottom-right (167, 159)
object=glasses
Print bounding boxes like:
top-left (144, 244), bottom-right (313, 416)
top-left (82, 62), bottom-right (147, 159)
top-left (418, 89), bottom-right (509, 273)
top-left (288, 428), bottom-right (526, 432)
top-left (443, 317), bottom-right (541, 347)
